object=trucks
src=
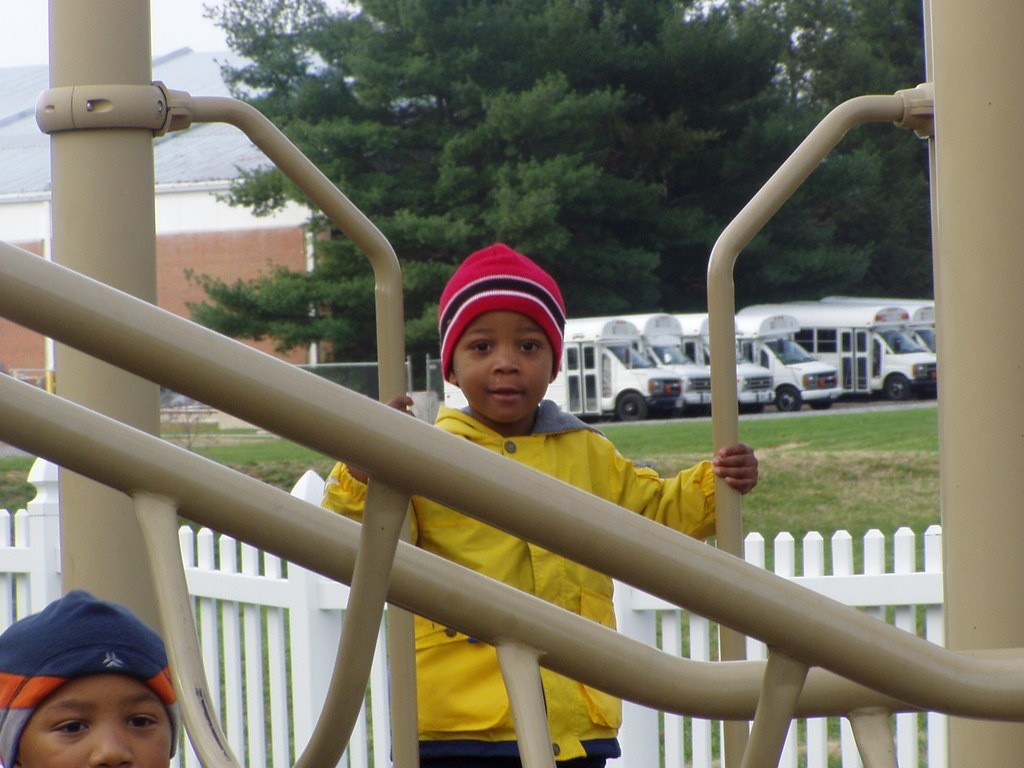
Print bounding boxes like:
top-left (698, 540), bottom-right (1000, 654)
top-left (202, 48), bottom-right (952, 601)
top-left (736, 297), bottom-right (937, 401)
top-left (443, 313), bottom-right (845, 422)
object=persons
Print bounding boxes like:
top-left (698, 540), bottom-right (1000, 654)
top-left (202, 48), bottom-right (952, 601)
top-left (322, 242), bottom-right (759, 768)
top-left (1, 590), bottom-right (180, 768)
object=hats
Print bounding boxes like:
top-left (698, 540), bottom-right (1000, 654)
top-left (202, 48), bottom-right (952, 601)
top-left (437, 242), bottom-right (566, 382)
top-left (0, 589), bottom-right (180, 768)
